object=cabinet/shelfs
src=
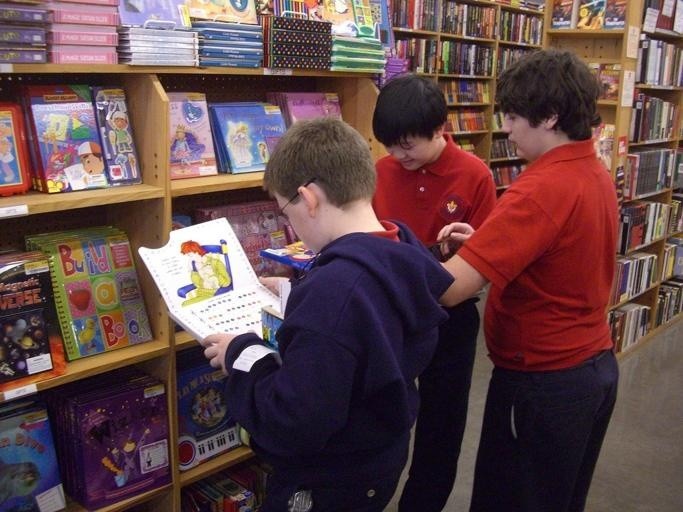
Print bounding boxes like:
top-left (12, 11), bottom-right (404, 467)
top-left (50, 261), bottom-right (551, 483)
top-left (1, 60), bottom-right (391, 511)
top-left (534, 1), bottom-right (683, 358)
top-left (383, 1), bottom-right (536, 198)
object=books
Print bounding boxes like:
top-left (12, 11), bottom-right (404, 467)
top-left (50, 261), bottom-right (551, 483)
top-left (1, 367), bottom-right (174, 510)
top-left (0, 1), bottom-right (387, 74)
top-left (1, 226), bottom-right (153, 384)
top-left (369, 1), bottom-right (545, 201)
top-left (165, 91), bottom-right (343, 180)
top-left (0, 80), bottom-right (143, 197)
top-left (177, 351), bottom-right (274, 510)
top-left (137, 193), bottom-right (319, 366)
top-left (552, 0), bottom-right (682, 355)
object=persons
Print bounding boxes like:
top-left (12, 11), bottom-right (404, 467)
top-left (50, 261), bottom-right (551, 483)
top-left (437, 48), bottom-right (619, 511)
top-left (202, 113), bottom-right (455, 510)
top-left (371, 73), bottom-right (498, 510)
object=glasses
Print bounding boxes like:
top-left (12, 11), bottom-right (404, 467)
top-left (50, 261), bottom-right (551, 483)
top-left (275, 175), bottom-right (317, 228)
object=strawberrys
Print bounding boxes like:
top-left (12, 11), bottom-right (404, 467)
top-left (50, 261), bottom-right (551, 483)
top-left (70, 282), bottom-right (91, 312)
